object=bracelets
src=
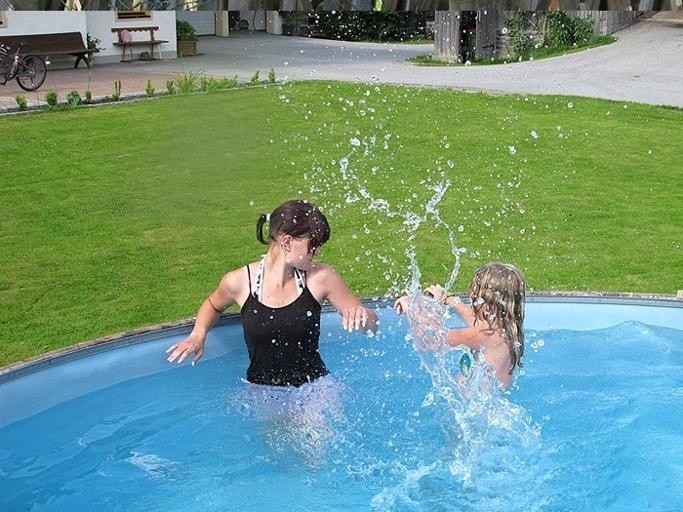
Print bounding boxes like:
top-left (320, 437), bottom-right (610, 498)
top-left (442, 294), bottom-right (455, 305)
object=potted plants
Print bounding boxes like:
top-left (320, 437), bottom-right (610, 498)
top-left (177, 21), bottom-right (196, 56)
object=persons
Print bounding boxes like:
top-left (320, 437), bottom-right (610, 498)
top-left (165, 202), bottom-right (378, 388)
top-left (393, 262), bottom-right (526, 400)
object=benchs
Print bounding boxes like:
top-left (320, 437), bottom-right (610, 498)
top-left (112, 27), bottom-right (162, 62)
top-left (0, 32), bottom-right (93, 69)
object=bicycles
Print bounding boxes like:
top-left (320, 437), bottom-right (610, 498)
top-left (0, 42), bottom-right (47, 93)
